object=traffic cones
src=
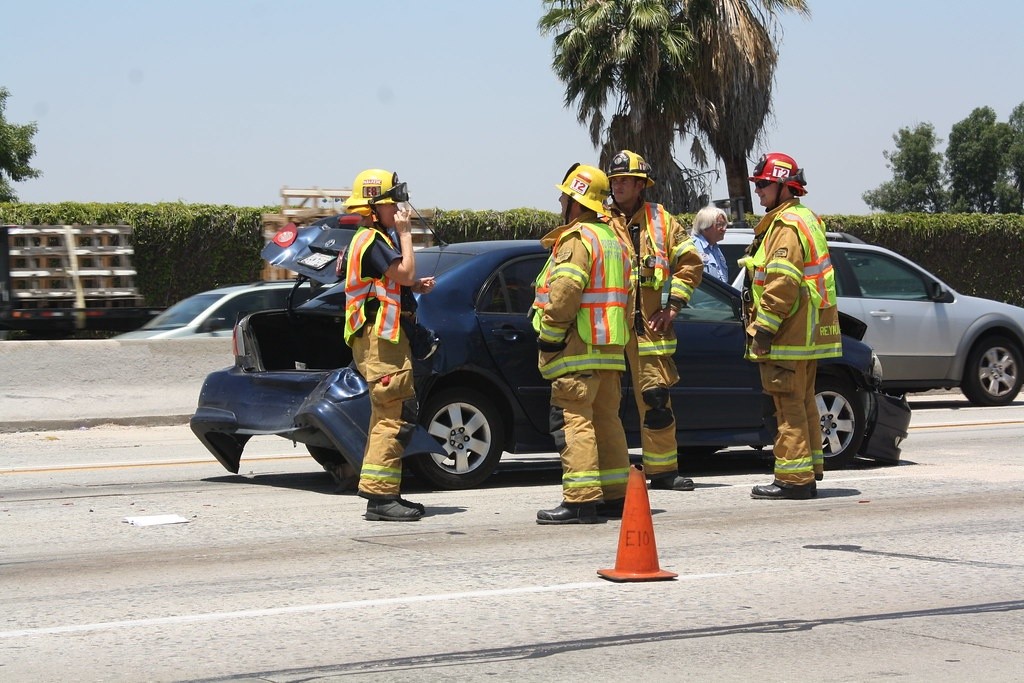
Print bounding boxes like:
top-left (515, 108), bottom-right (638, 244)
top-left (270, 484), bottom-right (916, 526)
top-left (596, 464), bottom-right (678, 581)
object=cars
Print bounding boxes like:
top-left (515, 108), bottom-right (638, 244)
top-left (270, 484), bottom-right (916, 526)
top-left (189, 238), bottom-right (911, 491)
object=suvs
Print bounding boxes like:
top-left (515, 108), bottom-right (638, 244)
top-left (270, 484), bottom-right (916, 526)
top-left (661, 228), bottom-right (1024, 407)
top-left (112, 280), bottom-right (338, 340)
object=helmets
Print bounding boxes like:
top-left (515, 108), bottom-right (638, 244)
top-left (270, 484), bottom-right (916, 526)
top-left (747, 153), bottom-right (808, 197)
top-left (342, 169), bottom-right (397, 205)
top-left (607, 150), bottom-right (655, 188)
top-left (556, 165), bottom-right (613, 218)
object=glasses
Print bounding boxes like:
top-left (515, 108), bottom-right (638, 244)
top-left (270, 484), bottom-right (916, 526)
top-left (755, 180), bottom-right (775, 188)
top-left (714, 221), bottom-right (728, 230)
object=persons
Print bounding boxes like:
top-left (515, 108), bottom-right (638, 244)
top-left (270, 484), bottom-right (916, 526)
top-left (737, 153), bottom-right (842, 501)
top-left (691, 206), bottom-right (731, 282)
top-left (342, 168), bottom-right (438, 522)
top-left (606, 151), bottom-right (708, 491)
top-left (527, 162), bottom-right (634, 525)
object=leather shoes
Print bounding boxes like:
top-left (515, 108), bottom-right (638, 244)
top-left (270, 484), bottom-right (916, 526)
top-left (536, 502), bottom-right (598, 524)
top-left (750, 483), bottom-right (817, 498)
top-left (395, 495), bottom-right (425, 515)
top-left (651, 473), bottom-right (694, 491)
top-left (366, 499), bottom-right (420, 521)
top-left (595, 497), bottom-right (625, 517)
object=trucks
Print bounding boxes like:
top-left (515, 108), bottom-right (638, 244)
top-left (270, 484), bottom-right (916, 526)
top-left (0, 224), bottom-right (167, 333)
